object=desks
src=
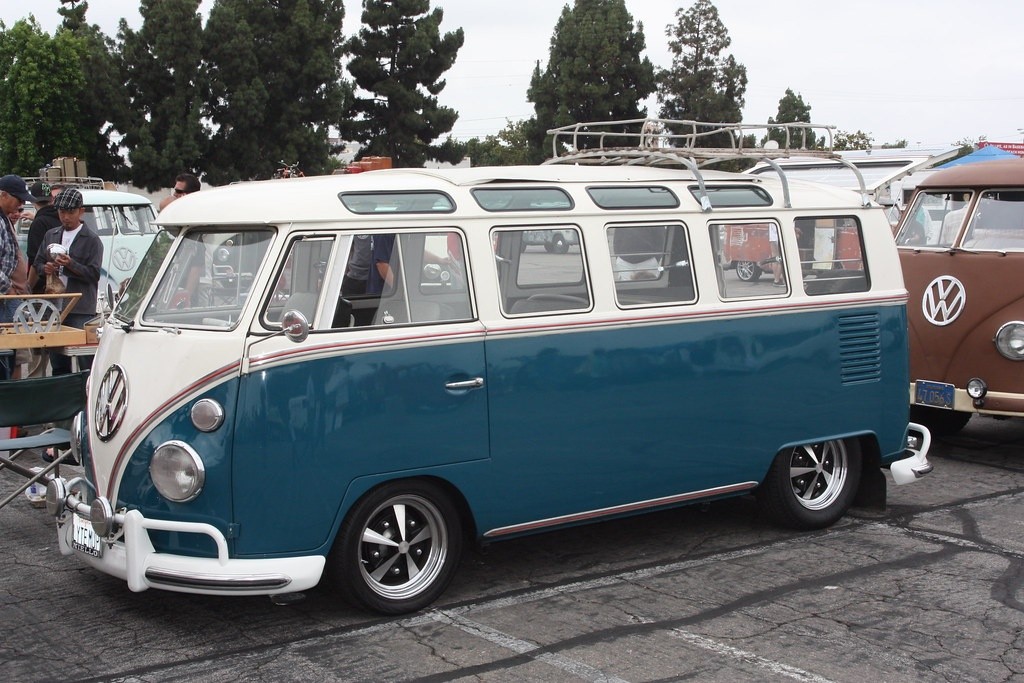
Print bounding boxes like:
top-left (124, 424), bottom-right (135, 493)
top-left (0, 350), bottom-right (15, 380)
top-left (41, 345), bottom-right (97, 378)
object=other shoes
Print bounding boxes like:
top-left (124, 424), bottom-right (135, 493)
top-left (17, 428), bottom-right (28, 437)
top-left (42, 447), bottom-right (80, 466)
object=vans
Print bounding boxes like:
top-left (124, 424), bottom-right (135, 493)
top-left (884, 158), bottom-right (1023, 435)
top-left (515, 149), bottom-right (930, 282)
top-left (0, 176), bottom-right (171, 323)
top-left (46, 117), bottom-right (935, 621)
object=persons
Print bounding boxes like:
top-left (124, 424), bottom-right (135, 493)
top-left (0, 174), bottom-right (668, 468)
top-left (768, 223), bottom-right (807, 288)
top-left (892, 192), bottom-right (973, 248)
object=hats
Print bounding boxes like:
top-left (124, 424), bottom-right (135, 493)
top-left (0, 174), bottom-right (29, 199)
top-left (31, 182), bottom-right (52, 202)
top-left (54, 188), bottom-right (83, 211)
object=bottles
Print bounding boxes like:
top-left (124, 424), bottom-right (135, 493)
top-left (29, 468), bottom-right (38, 495)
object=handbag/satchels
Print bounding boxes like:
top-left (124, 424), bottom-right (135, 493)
top-left (44, 272), bottom-right (64, 294)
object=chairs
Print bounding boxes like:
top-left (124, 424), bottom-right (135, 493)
top-left (0, 368), bottom-right (90, 510)
top-left (280, 291), bottom-right (354, 332)
top-left (507, 292), bottom-right (589, 316)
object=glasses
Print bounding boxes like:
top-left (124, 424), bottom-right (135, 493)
top-left (175, 189), bottom-right (186, 194)
top-left (18, 208), bottom-right (24, 213)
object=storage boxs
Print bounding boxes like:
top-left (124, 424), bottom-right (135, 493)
top-left (0, 292), bottom-right (87, 349)
top-left (84, 313), bottom-right (109, 342)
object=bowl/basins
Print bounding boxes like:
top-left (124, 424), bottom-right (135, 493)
top-left (47, 243), bottom-right (67, 261)
